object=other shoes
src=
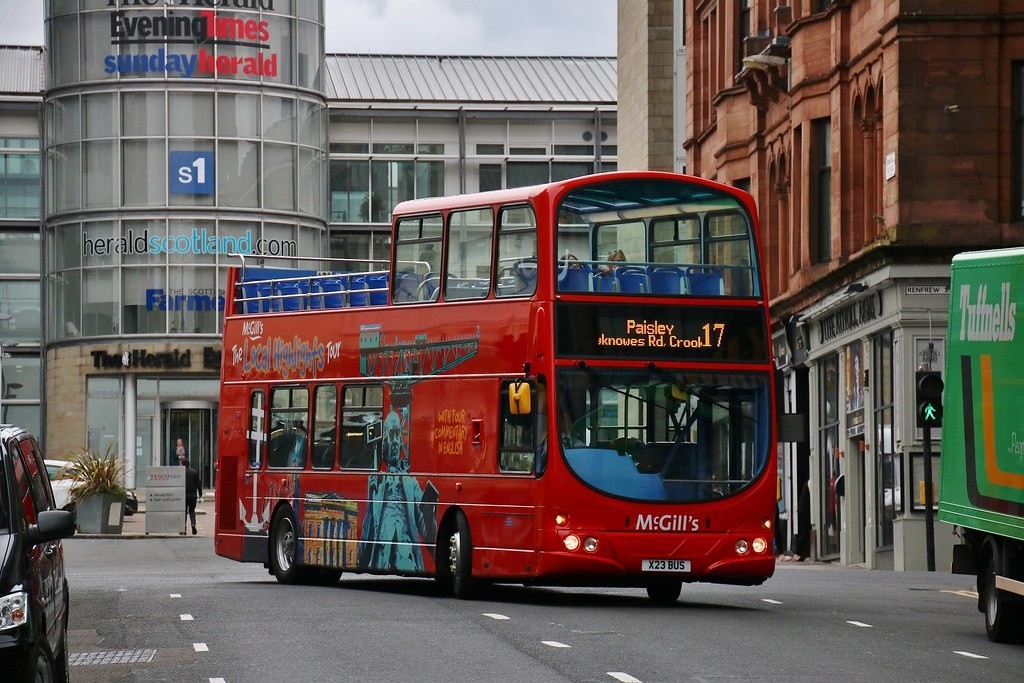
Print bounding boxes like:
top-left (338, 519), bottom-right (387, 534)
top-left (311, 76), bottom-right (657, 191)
top-left (178, 532), bottom-right (186, 535)
top-left (192, 525), bottom-right (197, 535)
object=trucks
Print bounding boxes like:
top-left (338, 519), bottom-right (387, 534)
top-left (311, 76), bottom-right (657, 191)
top-left (935, 245), bottom-right (1024, 646)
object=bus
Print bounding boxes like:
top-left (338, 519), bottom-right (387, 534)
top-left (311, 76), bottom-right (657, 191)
top-left (209, 170), bottom-right (804, 605)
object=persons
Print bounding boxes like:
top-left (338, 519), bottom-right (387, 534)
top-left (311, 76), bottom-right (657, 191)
top-left (357, 412), bottom-right (438, 572)
top-left (542, 411), bottom-right (584, 456)
top-left (561, 254), bottom-right (583, 268)
top-left (602, 249), bottom-right (627, 277)
top-left (179, 460), bottom-right (203, 535)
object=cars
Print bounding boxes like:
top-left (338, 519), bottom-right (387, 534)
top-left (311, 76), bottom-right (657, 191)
top-left (122, 488), bottom-right (138, 516)
top-left (42, 460), bottom-right (88, 530)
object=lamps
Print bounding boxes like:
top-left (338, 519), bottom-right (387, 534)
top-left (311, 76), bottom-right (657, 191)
top-left (742, 55), bottom-right (792, 92)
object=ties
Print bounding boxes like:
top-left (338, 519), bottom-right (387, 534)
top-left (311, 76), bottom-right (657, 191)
top-left (564, 437), bottom-right (571, 449)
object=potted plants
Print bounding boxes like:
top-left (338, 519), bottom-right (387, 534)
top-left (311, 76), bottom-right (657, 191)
top-left (559, 210), bottom-right (569, 223)
top-left (63, 439), bottom-right (134, 534)
top-left (359, 192), bottom-right (385, 222)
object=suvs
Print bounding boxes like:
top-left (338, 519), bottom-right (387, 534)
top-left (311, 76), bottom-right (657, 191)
top-left (0, 421), bottom-right (84, 683)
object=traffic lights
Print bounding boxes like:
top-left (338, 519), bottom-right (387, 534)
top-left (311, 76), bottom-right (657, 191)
top-left (915, 371), bottom-right (944, 427)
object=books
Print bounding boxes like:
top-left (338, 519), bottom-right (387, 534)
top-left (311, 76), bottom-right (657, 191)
top-left (418, 481), bottom-right (439, 513)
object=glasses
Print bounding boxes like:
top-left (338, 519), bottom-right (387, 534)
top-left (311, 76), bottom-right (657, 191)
top-left (608, 249), bottom-right (625, 256)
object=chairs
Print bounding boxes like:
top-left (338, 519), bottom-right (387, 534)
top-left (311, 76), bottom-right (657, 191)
top-left (272, 442), bottom-right (382, 469)
top-left (239, 259), bottom-right (725, 313)
top-left (610, 437), bottom-right (645, 463)
